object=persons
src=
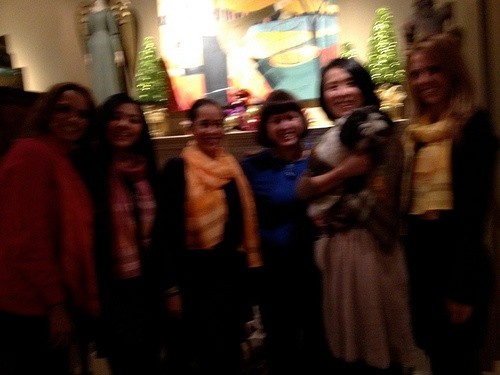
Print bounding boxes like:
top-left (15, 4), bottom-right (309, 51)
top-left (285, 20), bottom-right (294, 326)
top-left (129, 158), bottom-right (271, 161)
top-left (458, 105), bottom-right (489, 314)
top-left (293, 57), bottom-right (422, 375)
top-left (163, 96), bottom-right (264, 375)
top-left (0, 80), bottom-right (96, 375)
top-left (69, 92), bottom-right (172, 375)
top-left (233, 89), bottom-right (342, 375)
top-left (396, 32), bottom-right (499, 375)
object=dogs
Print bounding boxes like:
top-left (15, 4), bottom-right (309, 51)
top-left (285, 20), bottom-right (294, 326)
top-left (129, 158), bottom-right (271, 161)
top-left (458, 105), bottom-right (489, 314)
top-left (306, 107), bottom-right (394, 218)
